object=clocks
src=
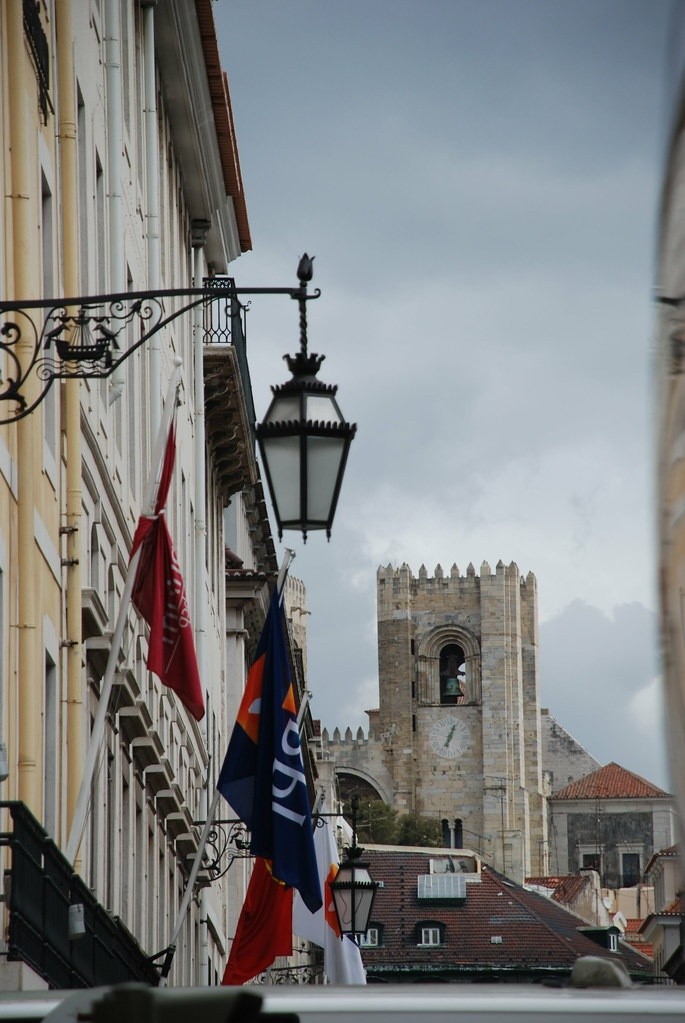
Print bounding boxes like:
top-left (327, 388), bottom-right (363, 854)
top-left (429, 717), bottom-right (470, 759)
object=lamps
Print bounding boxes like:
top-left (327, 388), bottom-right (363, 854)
top-left (0, 252), bottom-right (356, 544)
top-left (193, 794), bottom-right (379, 941)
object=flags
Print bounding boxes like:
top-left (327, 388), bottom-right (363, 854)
top-left (217, 569), bottom-right (324, 914)
top-left (128, 357), bottom-right (205, 722)
top-left (220, 784), bottom-right (367, 987)
